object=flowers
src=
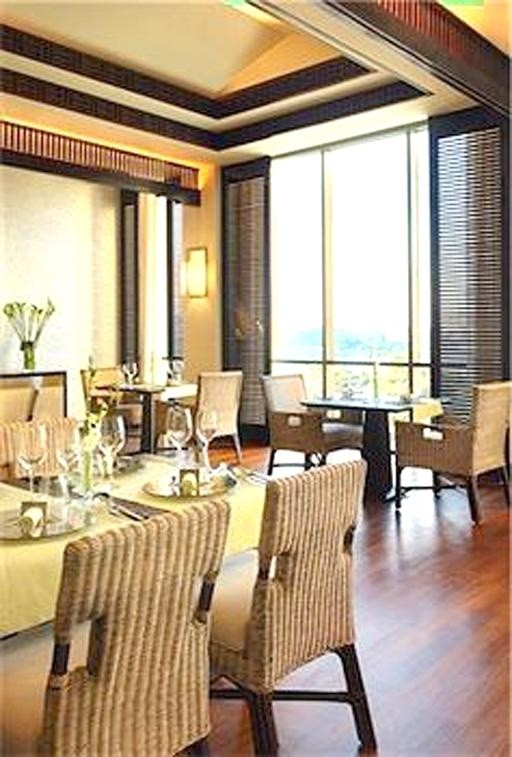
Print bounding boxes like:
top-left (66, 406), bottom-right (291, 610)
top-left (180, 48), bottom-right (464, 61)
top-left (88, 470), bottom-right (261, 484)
top-left (4, 296), bottom-right (56, 346)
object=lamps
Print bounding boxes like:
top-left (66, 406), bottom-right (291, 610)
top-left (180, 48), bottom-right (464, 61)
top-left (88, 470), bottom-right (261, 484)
top-left (183, 244), bottom-right (209, 299)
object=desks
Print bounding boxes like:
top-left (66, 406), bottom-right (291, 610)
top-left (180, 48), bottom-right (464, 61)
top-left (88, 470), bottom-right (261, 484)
top-left (1, 441), bottom-right (281, 642)
top-left (298, 391), bottom-right (448, 507)
top-left (98, 381), bottom-right (204, 462)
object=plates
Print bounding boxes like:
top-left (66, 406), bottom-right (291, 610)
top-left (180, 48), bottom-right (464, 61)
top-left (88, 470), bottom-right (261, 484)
top-left (2, 504), bottom-right (96, 543)
top-left (144, 466), bottom-right (240, 496)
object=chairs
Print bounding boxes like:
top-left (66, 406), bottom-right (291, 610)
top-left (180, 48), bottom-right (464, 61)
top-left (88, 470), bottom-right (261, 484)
top-left (193, 450), bottom-right (379, 755)
top-left (164, 370), bottom-right (259, 466)
top-left (386, 375), bottom-right (509, 527)
top-left (79, 362), bottom-right (147, 451)
top-left (259, 373), bottom-right (362, 478)
top-left (6, 411), bottom-right (87, 484)
top-left (1, 498), bottom-right (233, 756)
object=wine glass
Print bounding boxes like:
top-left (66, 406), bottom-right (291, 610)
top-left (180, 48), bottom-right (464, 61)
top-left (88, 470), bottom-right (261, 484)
top-left (9, 405), bottom-right (221, 499)
top-left (122, 356), bottom-right (182, 386)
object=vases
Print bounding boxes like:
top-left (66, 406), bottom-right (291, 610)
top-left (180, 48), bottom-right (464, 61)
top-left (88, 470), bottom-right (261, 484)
top-left (19, 341), bottom-right (37, 371)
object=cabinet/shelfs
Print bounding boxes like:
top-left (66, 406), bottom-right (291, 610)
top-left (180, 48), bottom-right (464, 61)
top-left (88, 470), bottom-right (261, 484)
top-left (0, 372), bottom-right (68, 446)
